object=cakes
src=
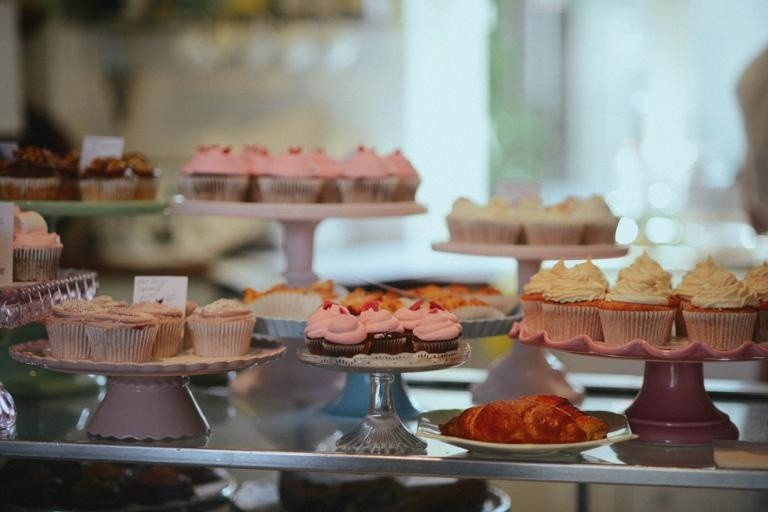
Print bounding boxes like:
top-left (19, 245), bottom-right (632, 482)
top-left (0, 202), bottom-right (62, 280)
top-left (302, 298), bottom-right (463, 354)
top-left (1, 144), bottom-right (159, 200)
top-left (277, 468), bottom-right (485, 512)
top-left (0, 459), bottom-right (216, 511)
top-left (48, 295), bottom-right (258, 366)
top-left (521, 255), bottom-right (768, 342)
top-left (183, 139), bottom-right (422, 202)
top-left (448, 192), bottom-right (621, 245)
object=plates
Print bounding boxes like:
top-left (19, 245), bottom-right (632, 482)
top-left (414, 405), bottom-right (639, 459)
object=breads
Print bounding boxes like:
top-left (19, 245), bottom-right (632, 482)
top-left (436, 394), bottom-right (609, 442)
top-left (342, 284), bottom-right (504, 311)
top-left (711, 438), bottom-right (768, 469)
top-left (240, 280), bottom-right (334, 303)
